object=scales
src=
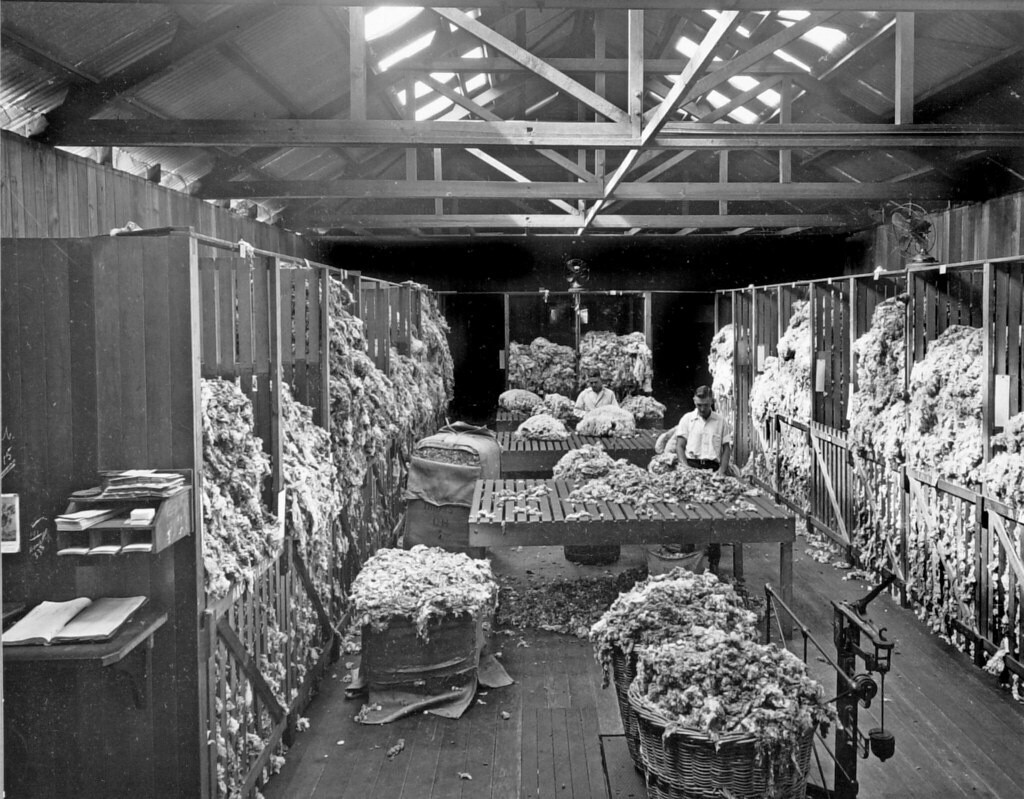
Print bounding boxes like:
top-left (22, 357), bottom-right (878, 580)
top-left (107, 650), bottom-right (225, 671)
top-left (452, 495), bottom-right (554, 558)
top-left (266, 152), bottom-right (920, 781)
top-left (597, 572), bottom-right (900, 799)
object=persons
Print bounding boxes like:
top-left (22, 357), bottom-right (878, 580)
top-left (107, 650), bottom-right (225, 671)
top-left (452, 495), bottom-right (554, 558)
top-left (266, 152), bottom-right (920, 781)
top-left (676, 385), bottom-right (735, 579)
top-left (573, 369), bottom-right (620, 437)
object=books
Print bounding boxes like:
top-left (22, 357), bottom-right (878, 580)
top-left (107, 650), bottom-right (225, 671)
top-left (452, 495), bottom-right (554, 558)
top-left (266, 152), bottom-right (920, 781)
top-left (55, 505), bottom-right (131, 531)
top-left (100, 466), bottom-right (185, 497)
top-left (2, 597), bottom-right (148, 646)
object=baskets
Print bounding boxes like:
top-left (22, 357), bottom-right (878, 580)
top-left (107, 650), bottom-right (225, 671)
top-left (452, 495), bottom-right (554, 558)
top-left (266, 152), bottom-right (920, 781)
top-left (606, 643), bottom-right (649, 772)
top-left (626, 673), bottom-right (819, 799)
top-left (564, 539), bottom-right (620, 566)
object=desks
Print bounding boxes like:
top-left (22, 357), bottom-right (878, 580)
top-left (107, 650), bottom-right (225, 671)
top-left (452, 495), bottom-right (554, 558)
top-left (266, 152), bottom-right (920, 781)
top-left (494, 430), bottom-right (672, 478)
top-left (467, 477), bottom-right (796, 640)
top-left (495, 406), bottom-right (664, 430)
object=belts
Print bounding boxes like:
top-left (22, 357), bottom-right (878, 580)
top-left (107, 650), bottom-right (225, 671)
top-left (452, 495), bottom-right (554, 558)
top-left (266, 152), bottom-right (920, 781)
top-left (689, 458), bottom-right (715, 465)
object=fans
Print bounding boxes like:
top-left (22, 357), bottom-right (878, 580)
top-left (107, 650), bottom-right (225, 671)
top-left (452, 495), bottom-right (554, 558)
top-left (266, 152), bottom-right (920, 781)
top-left (887, 202), bottom-right (940, 271)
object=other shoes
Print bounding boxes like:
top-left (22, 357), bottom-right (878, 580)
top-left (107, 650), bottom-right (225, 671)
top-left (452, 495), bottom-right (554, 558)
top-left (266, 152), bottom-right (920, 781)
top-left (709, 561), bottom-right (718, 575)
top-left (680, 544), bottom-right (696, 553)
